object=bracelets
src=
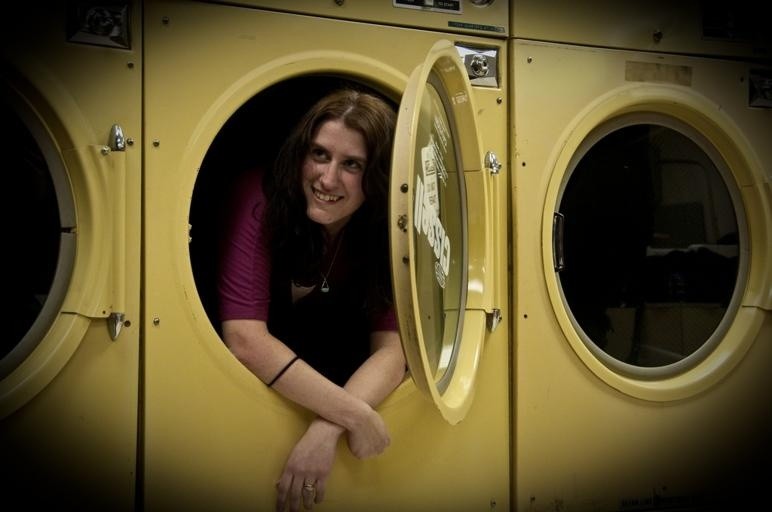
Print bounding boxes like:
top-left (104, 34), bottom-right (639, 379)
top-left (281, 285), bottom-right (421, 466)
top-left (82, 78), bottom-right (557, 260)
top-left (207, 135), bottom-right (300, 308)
top-left (267, 356), bottom-right (302, 388)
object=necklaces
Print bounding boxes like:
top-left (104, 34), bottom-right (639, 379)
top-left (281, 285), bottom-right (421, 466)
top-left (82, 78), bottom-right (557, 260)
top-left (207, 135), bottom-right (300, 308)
top-left (309, 224), bottom-right (346, 293)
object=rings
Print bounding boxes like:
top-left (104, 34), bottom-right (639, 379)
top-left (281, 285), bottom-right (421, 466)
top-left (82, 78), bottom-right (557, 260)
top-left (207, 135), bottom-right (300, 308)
top-left (303, 479), bottom-right (317, 494)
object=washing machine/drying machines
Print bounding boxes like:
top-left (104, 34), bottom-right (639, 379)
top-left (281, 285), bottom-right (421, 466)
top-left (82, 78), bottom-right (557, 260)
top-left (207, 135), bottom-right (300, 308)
top-left (510, 0), bottom-right (770, 512)
top-left (143, 2), bottom-right (511, 512)
top-left (3, 2), bottom-right (143, 512)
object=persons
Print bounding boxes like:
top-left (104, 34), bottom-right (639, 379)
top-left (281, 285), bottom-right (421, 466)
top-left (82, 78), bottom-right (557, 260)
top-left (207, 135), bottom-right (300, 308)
top-left (216, 90), bottom-right (407, 512)
